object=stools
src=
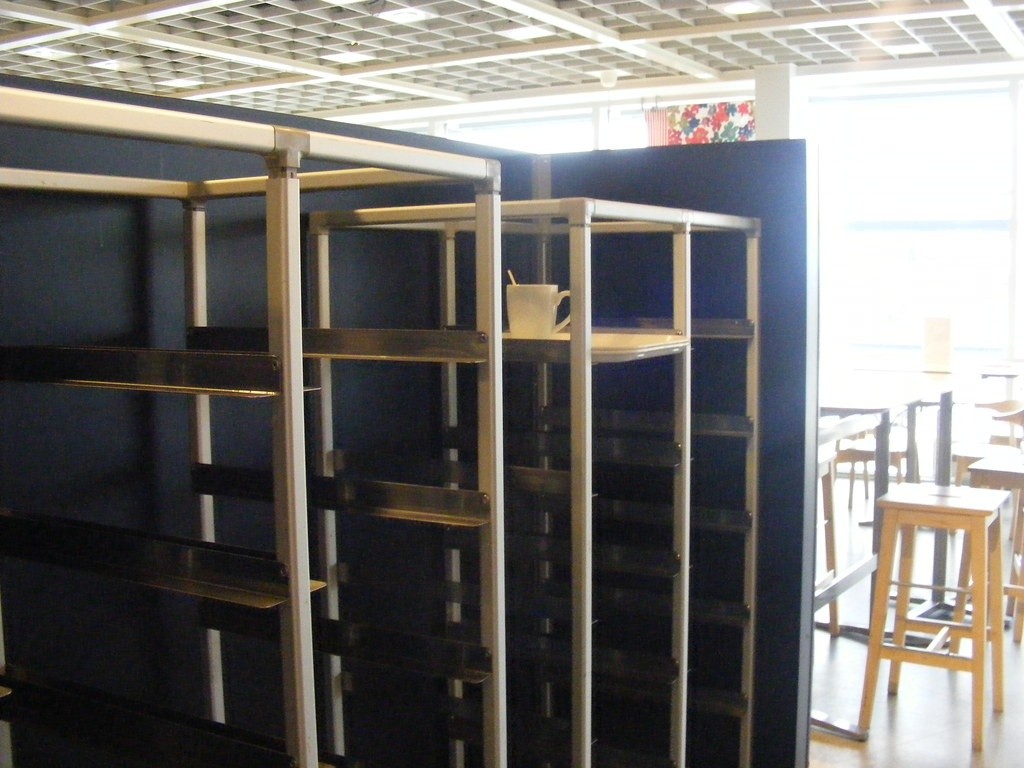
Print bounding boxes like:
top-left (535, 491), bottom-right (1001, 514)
top-left (814, 457), bottom-right (841, 644)
top-left (853, 481), bottom-right (1012, 752)
top-left (951, 451), bottom-right (1024, 655)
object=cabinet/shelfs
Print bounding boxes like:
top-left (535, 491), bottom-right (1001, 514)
top-left (302, 196), bottom-right (761, 768)
top-left (2, 87), bottom-right (511, 768)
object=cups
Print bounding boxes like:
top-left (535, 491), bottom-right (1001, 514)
top-left (506, 284), bottom-right (572, 339)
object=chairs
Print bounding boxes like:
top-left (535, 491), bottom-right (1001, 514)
top-left (950, 399), bottom-right (1023, 531)
top-left (832, 406), bottom-right (910, 515)
